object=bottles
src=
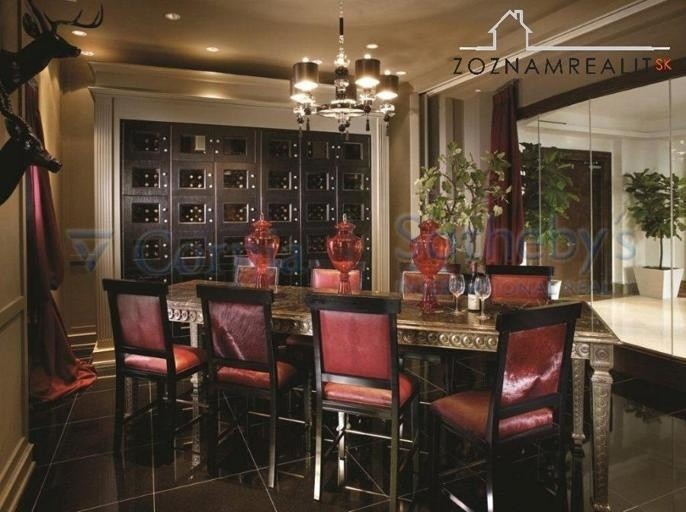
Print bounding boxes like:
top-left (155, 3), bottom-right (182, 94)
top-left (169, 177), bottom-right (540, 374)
top-left (244, 212), bottom-right (281, 290)
top-left (465, 259), bottom-right (481, 313)
top-left (326, 212), bottom-right (364, 295)
top-left (408, 219), bottom-right (449, 314)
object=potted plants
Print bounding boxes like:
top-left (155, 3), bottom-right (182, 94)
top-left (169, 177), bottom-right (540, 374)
top-left (621, 167), bottom-right (685, 301)
top-left (414, 140), bottom-right (512, 295)
top-left (517, 139), bottom-right (580, 301)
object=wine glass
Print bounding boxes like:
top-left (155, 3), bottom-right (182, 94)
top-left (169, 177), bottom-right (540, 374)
top-left (447, 273), bottom-right (465, 316)
top-left (474, 276), bottom-right (492, 321)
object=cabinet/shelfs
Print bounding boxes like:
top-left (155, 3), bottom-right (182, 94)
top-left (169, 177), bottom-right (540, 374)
top-left (118, 119), bottom-right (373, 346)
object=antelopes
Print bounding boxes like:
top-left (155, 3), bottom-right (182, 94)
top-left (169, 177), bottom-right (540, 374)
top-left (0, 88), bottom-right (63, 207)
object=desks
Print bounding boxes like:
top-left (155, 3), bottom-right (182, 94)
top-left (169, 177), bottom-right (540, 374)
top-left (157, 279), bottom-right (623, 512)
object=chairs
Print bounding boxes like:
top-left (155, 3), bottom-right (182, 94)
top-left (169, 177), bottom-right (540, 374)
top-left (304, 296), bottom-right (420, 512)
top-left (233, 256), bottom-right (283, 287)
top-left (427, 304), bottom-right (583, 512)
top-left (483, 265), bottom-right (554, 315)
top-left (195, 284), bottom-right (314, 489)
top-left (400, 262), bottom-right (461, 295)
top-left (102, 277), bottom-right (209, 465)
top-left (308, 257), bottom-right (366, 292)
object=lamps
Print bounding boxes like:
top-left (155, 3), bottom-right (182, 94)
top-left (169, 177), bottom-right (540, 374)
top-left (289, 1), bottom-right (399, 141)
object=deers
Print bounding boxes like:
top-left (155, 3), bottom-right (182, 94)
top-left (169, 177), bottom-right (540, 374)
top-left (0, 0), bottom-right (104, 96)
